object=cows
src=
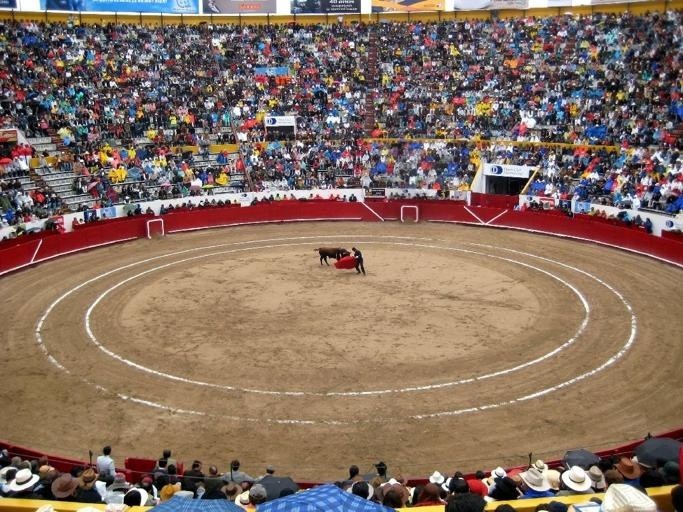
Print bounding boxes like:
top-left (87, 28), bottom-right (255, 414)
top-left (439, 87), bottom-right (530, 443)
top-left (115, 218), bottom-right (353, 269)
top-left (313, 247), bottom-right (352, 266)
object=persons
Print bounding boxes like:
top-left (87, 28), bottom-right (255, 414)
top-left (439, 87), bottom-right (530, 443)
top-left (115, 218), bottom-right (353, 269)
top-left (352, 247), bottom-right (366, 276)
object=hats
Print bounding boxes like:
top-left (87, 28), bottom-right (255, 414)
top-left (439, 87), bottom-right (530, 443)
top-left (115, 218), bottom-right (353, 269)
top-left (160, 484), bottom-right (181, 503)
top-left (106, 472), bottom-right (153, 506)
top-left (1, 455), bottom-right (99, 498)
top-left (345, 457), bottom-right (679, 512)
top-left (220, 483), bottom-right (268, 509)
